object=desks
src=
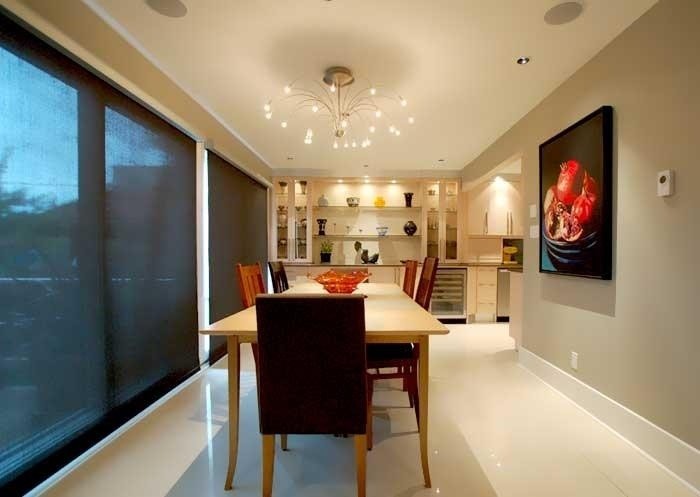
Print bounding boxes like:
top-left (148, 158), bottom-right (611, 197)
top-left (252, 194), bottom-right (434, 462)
top-left (198, 278), bottom-right (450, 490)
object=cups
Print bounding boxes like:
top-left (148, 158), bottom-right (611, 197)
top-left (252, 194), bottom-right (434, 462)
top-left (404, 192), bottom-right (414, 208)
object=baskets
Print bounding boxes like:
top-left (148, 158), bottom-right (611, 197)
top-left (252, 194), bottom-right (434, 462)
top-left (314, 269), bottom-right (369, 293)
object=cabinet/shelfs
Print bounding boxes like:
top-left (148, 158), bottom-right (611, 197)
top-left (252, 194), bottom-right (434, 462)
top-left (273, 175), bottom-right (524, 323)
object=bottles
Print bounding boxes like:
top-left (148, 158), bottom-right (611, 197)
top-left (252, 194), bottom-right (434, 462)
top-left (318, 194), bottom-right (328, 206)
top-left (404, 221), bottom-right (417, 235)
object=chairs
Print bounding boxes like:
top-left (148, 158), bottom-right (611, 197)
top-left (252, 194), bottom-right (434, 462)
top-left (252, 291), bottom-right (376, 497)
top-left (234, 261), bottom-right (291, 368)
top-left (372, 256), bottom-right (439, 434)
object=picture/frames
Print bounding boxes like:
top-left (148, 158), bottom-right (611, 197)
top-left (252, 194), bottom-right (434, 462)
top-left (539, 106), bottom-right (613, 280)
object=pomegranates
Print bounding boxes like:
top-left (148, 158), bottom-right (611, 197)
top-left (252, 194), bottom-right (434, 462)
top-left (543, 160), bottom-right (602, 243)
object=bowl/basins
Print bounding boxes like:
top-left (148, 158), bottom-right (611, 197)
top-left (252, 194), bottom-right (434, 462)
top-left (376, 226), bottom-right (388, 235)
top-left (346, 197), bottom-right (360, 206)
